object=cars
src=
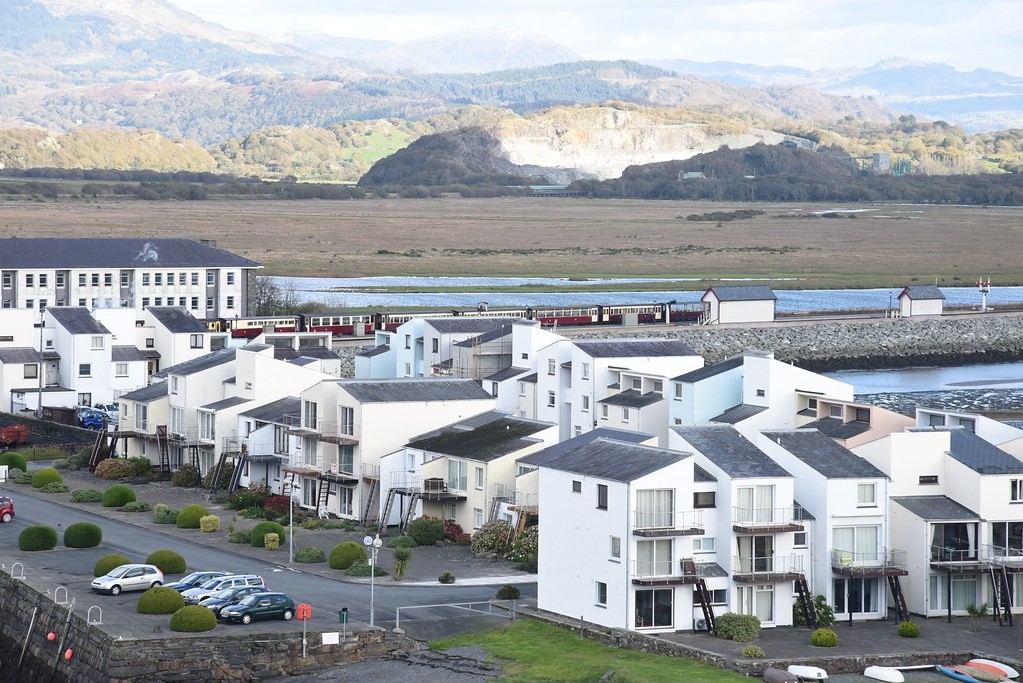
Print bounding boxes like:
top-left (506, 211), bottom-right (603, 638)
top-left (163, 570), bottom-right (233, 594)
top-left (74, 400), bottom-right (124, 432)
top-left (198, 585), bottom-right (273, 621)
top-left (219, 590), bottom-right (297, 625)
top-left (0, 496), bottom-right (15, 524)
top-left (91, 564), bottom-right (165, 596)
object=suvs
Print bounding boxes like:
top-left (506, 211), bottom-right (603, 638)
top-left (180, 572), bottom-right (267, 605)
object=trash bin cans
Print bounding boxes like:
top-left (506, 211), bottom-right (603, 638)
top-left (339, 610), bottom-right (348, 623)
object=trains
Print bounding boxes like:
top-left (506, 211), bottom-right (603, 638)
top-left (197, 288), bottom-right (709, 337)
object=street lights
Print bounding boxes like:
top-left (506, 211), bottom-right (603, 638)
top-left (364, 536), bottom-right (383, 628)
top-left (284, 478), bottom-right (300, 563)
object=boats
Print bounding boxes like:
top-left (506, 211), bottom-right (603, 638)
top-left (787, 663), bottom-right (829, 681)
top-left (864, 665), bottom-right (905, 683)
top-left (935, 664), bottom-right (982, 683)
top-left (763, 668), bottom-right (799, 683)
